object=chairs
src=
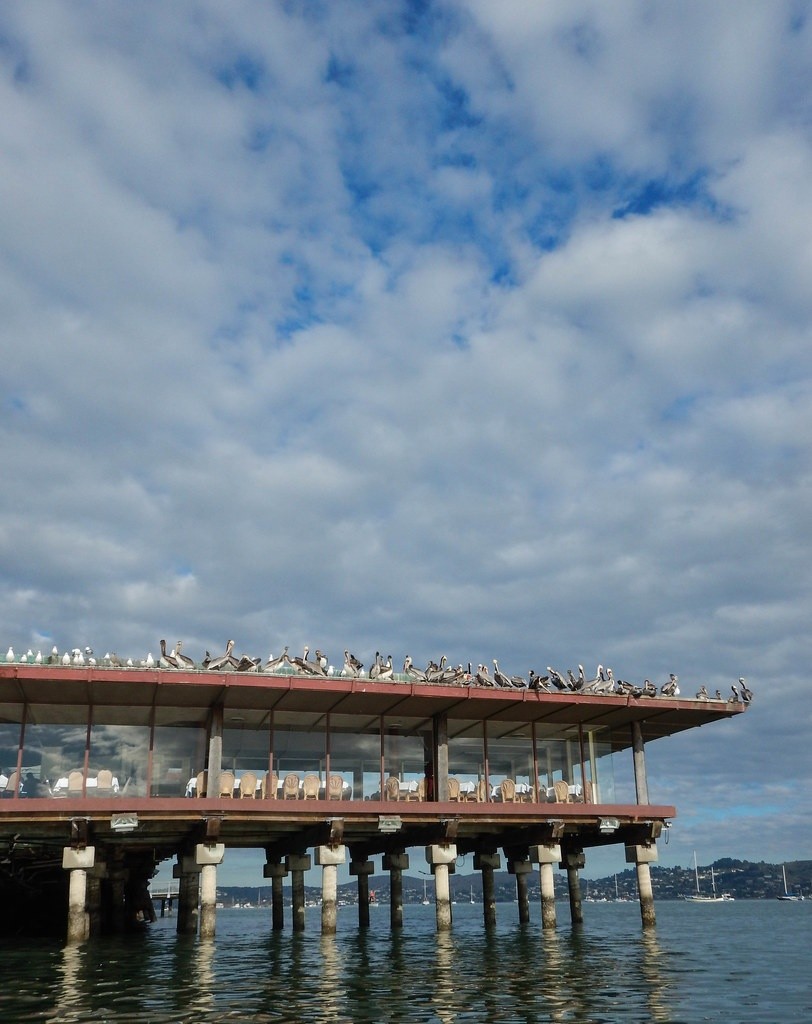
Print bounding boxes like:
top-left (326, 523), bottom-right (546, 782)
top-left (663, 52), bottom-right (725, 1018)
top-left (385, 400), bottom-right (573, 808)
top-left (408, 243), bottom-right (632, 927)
top-left (477, 780), bottom-right (485, 803)
top-left (500, 779), bottom-right (516, 803)
top-left (449, 778), bottom-right (460, 802)
top-left (118, 777), bottom-right (134, 798)
top-left (195, 770), bottom-right (209, 798)
top-left (282, 773), bottom-right (300, 800)
top-left (386, 776), bottom-right (400, 801)
top-left (553, 780), bottom-right (569, 803)
top-left (580, 780), bottom-right (592, 803)
top-left (218, 771), bottom-right (234, 799)
top-left (68, 771), bottom-right (83, 798)
top-left (406, 780), bottom-right (424, 802)
top-left (302, 774), bottom-right (320, 800)
top-left (44, 774), bottom-right (67, 798)
top-left (260, 773), bottom-right (278, 800)
top-left (238, 772), bottom-right (257, 800)
top-left (325, 775), bottom-right (343, 801)
top-left (96, 770), bottom-right (115, 798)
top-left (5, 771), bottom-right (17, 793)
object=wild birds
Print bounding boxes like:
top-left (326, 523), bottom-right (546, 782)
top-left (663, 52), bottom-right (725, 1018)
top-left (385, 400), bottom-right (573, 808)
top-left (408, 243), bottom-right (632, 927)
top-left (201, 638), bottom-right (261, 671)
top-left (158, 636), bottom-right (196, 670)
top-left (476, 658), bottom-right (528, 688)
top-left (616, 669), bottom-right (681, 700)
top-left (529, 664), bottom-right (615, 694)
top-left (263, 643), bottom-right (336, 677)
top-left (369, 651), bottom-right (395, 681)
top-left (695, 676), bottom-right (754, 705)
top-left (4, 645), bottom-right (156, 668)
top-left (340, 648), bottom-right (366, 678)
top-left (402, 653), bottom-right (473, 686)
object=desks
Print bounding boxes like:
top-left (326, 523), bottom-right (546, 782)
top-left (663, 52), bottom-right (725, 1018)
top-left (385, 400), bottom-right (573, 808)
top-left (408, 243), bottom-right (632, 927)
top-left (0, 774), bottom-right (24, 798)
top-left (184, 778), bottom-right (352, 801)
top-left (53, 777), bottom-right (119, 798)
top-left (378, 780), bottom-right (582, 804)
